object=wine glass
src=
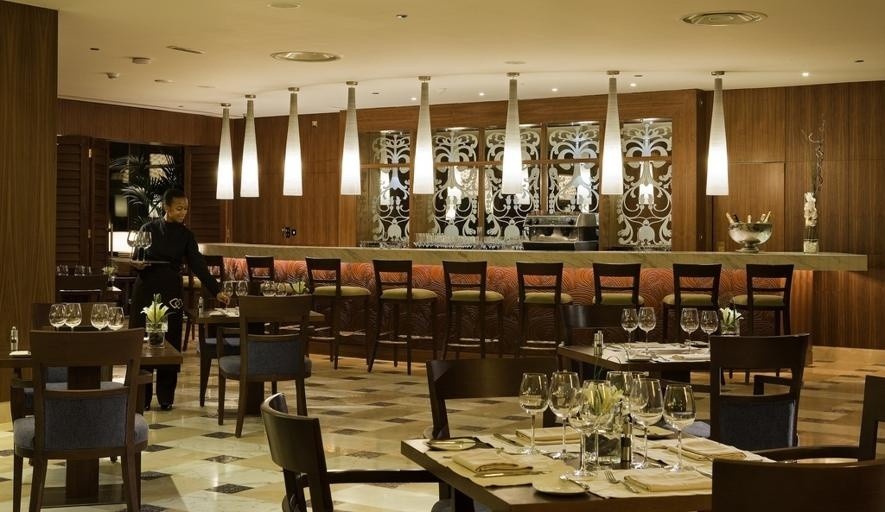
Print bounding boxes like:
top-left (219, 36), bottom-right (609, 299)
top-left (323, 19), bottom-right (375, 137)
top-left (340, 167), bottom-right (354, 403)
top-left (413, 232), bottom-right (525, 251)
top-left (728, 224), bottom-right (772, 253)
top-left (49, 302), bottom-right (125, 331)
top-left (621, 307), bottom-right (656, 352)
top-left (680, 308), bottom-right (719, 353)
top-left (519, 372), bottom-right (696, 472)
top-left (127, 229), bottom-right (152, 262)
top-left (221, 279), bottom-right (286, 313)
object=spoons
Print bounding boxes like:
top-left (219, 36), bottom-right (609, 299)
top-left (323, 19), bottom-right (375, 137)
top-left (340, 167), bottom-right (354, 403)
top-left (560, 474), bottom-right (589, 489)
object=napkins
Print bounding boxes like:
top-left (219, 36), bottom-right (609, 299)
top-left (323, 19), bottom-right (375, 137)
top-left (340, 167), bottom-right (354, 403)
top-left (515, 426), bottom-right (581, 445)
top-left (452, 449), bottom-right (534, 473)
top-left (668, 439), bottom-right (747, 461)
top-left (624, 470), bottom-right (712, 492)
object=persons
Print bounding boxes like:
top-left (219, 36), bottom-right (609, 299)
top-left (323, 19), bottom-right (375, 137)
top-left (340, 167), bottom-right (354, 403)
top-left (129, 189), bottom-right (230, 411)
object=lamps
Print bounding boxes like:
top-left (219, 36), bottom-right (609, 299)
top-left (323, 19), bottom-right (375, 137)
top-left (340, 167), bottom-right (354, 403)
top-left (411, 73), bottom-right (436, 194)
top-left (704, 69), bottom-right (731, 197)
top-left (337, 81), bottom-right (362, 196)
top-left (599, 70), bottom-right (627, 197)
top-left (380, 118), bottom-right (657, 219)
top-left (238, 94), bottom-right (260, 201)
top-left (499, 70), bottom-right (525, 197)
top-left (279, 86), bottom-right (304, 197)
top-left (215, 102), bottom-right (234, 201)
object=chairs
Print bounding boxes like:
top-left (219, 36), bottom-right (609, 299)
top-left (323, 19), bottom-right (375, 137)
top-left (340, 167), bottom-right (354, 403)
top-left (260, 392), bottom-right (475, 512)
top-left (198, 295), bottom-right (313, 439)
top-left (9, 274), bottom-right (145, 512)
top-left (709, 334), bottom-right (810, 449)
top-left (712, 459), bottom-right (884, 510)
top-left (746, 374), bottom-right (885, 463)
top-left (426, 355), bottom-right (564, 511)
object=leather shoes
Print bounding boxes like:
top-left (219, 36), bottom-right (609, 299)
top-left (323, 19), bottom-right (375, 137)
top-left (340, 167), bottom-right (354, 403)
top-left (161, 401), bottom-right (172, 410)
top-left (144, 404), bottom-right (151, 412)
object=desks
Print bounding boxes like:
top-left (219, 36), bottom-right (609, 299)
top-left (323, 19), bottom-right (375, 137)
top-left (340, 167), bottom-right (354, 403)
top-left (1, 333), bottom-right (184, 499)
top-left (401, 423), bottom-right (779, 512)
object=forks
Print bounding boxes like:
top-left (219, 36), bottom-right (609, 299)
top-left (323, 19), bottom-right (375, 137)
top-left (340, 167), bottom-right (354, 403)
top-left (605, 471), bottom-right (640, 493)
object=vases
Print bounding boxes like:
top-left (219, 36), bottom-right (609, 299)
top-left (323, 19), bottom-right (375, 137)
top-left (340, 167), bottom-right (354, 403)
top-left (804, 240), bottom-right (819, 253)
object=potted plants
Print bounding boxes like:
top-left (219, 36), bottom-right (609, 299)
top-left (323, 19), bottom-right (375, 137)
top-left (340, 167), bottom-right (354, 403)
top-left (140, 293), bottom-right (172, 349)
top-left (577, 380), bottom-right (632, 469)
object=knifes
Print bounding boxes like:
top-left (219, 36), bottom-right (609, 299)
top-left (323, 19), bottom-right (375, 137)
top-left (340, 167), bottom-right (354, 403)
top-left (474, 470), bottom-right (542, 476)
top-left (426, 439), bottom-right (484, 445)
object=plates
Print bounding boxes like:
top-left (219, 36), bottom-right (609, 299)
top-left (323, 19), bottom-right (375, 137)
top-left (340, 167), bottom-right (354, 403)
top-left (627, 355), bottom-right (652, 362)
top-left (8, 351), bottom-right (31, 357)
top-left (423, 439), bottom-right (476, 450)
top-left (532, 478), bottom-right (590, 495)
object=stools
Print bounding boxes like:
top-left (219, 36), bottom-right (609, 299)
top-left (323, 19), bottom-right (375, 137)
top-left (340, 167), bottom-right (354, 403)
top-left (130, 255), bottom-right (794, 376)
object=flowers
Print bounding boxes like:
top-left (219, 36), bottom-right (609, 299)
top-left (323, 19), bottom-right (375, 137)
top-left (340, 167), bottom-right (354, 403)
top-left (802, 191), bottom-right (821, 238)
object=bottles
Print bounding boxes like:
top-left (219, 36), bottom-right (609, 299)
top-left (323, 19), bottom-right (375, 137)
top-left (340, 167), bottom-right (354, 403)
top-left (10, 326), bottom-right (18, 352)
top-left (726, 211), bottom-right (771, 224)
top-left (57, 265), bottom-right (92, 277)
top-left (594, 331), bottom-right (603, 355)
top-left (198, 296), bottom-right (204, 314)
top-left (359, 240), bottom-right (379, 247)
